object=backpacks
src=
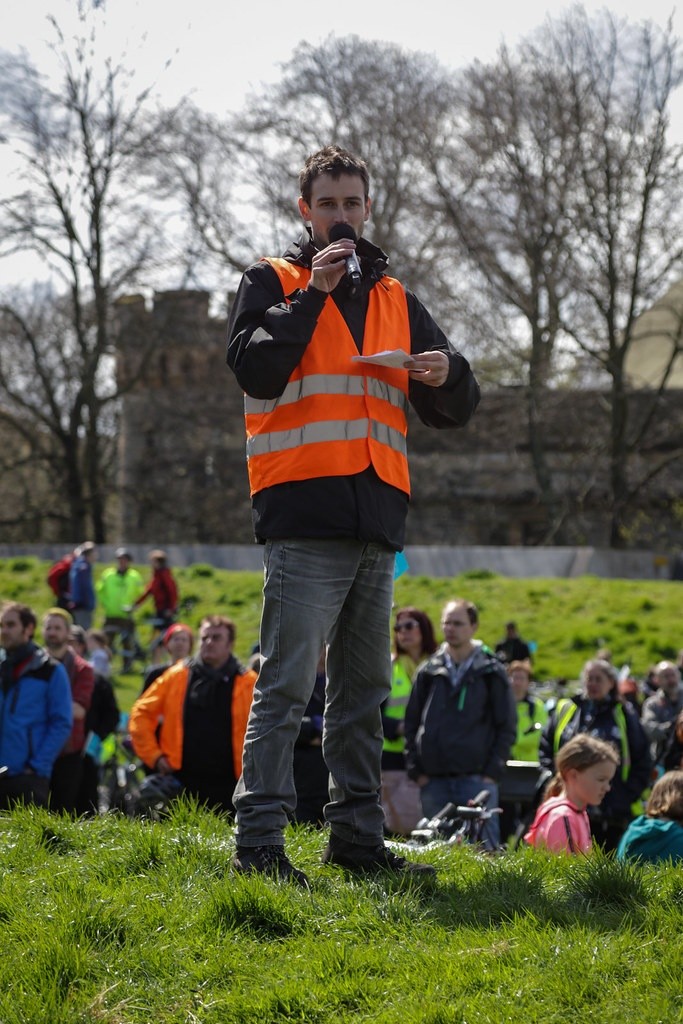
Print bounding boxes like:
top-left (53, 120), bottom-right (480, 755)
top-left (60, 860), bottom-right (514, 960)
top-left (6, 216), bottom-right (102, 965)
top-left (48, 557), bottom-right (74, 599)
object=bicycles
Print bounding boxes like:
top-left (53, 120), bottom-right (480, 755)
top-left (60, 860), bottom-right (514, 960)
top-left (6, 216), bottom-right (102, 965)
top-left (96, 608), bottom-right (172, 675)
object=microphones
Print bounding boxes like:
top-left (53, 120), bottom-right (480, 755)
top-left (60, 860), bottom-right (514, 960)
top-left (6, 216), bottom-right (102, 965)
top-left (329, 223), bottom-right (363, 285)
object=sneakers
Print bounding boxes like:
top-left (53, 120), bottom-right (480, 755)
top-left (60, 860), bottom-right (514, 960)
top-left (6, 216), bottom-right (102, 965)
top-left (232, 844), bottom-right (310, 888)
top-left (322, 832), bottom-right (436, 883)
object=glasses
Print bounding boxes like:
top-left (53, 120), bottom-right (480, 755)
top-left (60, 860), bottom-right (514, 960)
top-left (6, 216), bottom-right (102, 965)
top-left (394, 621), bottom-right (414, 631)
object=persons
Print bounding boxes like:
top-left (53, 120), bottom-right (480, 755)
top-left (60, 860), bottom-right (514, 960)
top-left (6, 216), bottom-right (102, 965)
top-left (124, 550), bottom-right (179, 646)
top-left (225, 145), bottom-right (482, 889)
top-left (380, 607), bottom-right (439, 771)
top-left (404, 599), bottom-right (518, 852)
top-left (506, 648), bottom-right (683, 853)
top-left (0, 601), bottom-right (72, 812)
top-left (40, 607), bottom-right (121, 823)
top-left (129, 615), bottom-right (259, 820)
top-left (142, 622), bottom-right (330, 832)
top-left (495, 622), bottom-right (530, 664)
top-left (47, 542), bottom-right (98, 632)
top-left (95, 547), bottom-right (147, 675)
top-left (616, 770), bottom-right (683, 870)
top-left (523, 733), bottom-right (619, 854)
